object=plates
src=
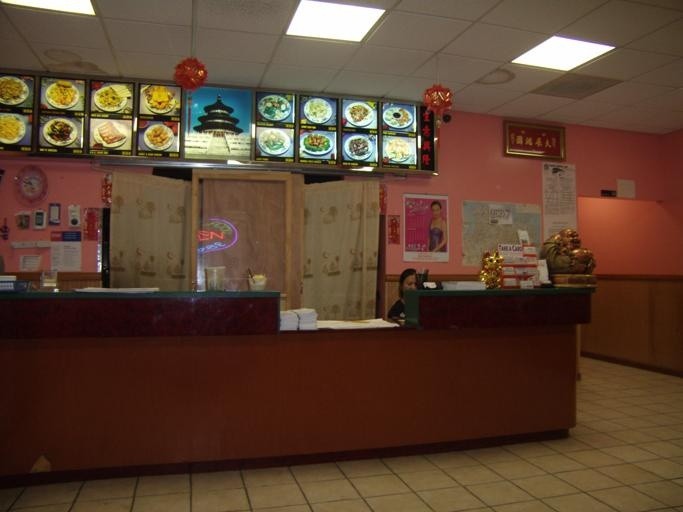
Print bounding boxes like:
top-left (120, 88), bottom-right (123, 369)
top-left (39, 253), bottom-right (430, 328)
top-left (0, 75), bottom-right (177, 152)
top-left (257, 94), bottom-right (414, 163)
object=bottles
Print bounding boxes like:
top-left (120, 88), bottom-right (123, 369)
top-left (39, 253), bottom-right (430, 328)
top-left (204, 266), bottom-right (226, 292)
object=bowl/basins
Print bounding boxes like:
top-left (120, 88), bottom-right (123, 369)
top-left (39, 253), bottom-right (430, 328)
top-left (248, 276), bottom-right (268, 291)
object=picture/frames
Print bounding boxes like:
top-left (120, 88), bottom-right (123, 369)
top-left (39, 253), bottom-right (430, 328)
top-left (503, 121), bottom-right (566, 162)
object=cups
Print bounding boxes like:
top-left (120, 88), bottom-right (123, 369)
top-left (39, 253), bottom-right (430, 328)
top-left (40, 265), bottom-right (58, 292)
top-left (415, 274), bottom-right (428, 290)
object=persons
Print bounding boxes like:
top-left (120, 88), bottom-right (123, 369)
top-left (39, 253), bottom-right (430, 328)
top-left (425, 201), bottom-right (447, 252)
top-left (388, 268), bottom-right (418, 321)
